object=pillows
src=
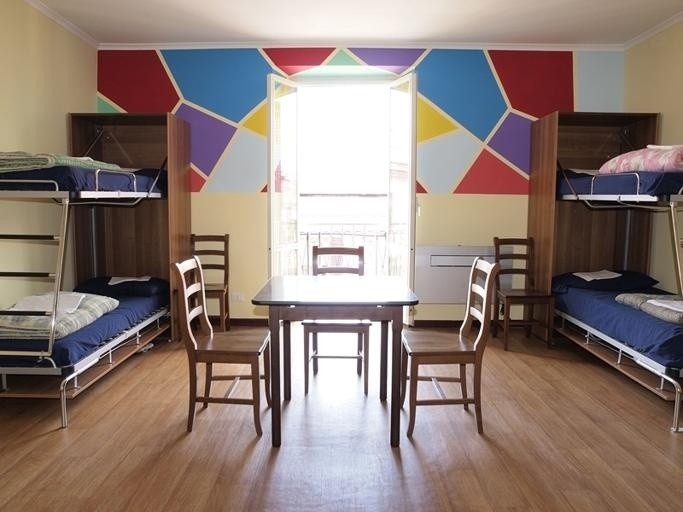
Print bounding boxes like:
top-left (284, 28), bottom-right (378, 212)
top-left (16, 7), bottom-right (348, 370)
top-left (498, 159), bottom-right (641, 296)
top-left (553, 269), bottom-right (660, 292)
top-left (72, 274), bottom-right (164, 298)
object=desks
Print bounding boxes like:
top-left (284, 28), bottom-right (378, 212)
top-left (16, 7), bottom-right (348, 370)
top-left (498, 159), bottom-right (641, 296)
top-left (251, 276), bottom-right (420, 447)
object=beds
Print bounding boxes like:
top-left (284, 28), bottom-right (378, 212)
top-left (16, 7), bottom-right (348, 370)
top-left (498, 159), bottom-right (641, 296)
top-left (551, 169), bottom-right (683, 431)
top-left (0, 149), bottom-right (170, 429)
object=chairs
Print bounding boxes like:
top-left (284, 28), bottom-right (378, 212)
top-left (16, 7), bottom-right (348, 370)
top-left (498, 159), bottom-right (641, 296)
top-left (493, 237), bottom-right (555, 353)
